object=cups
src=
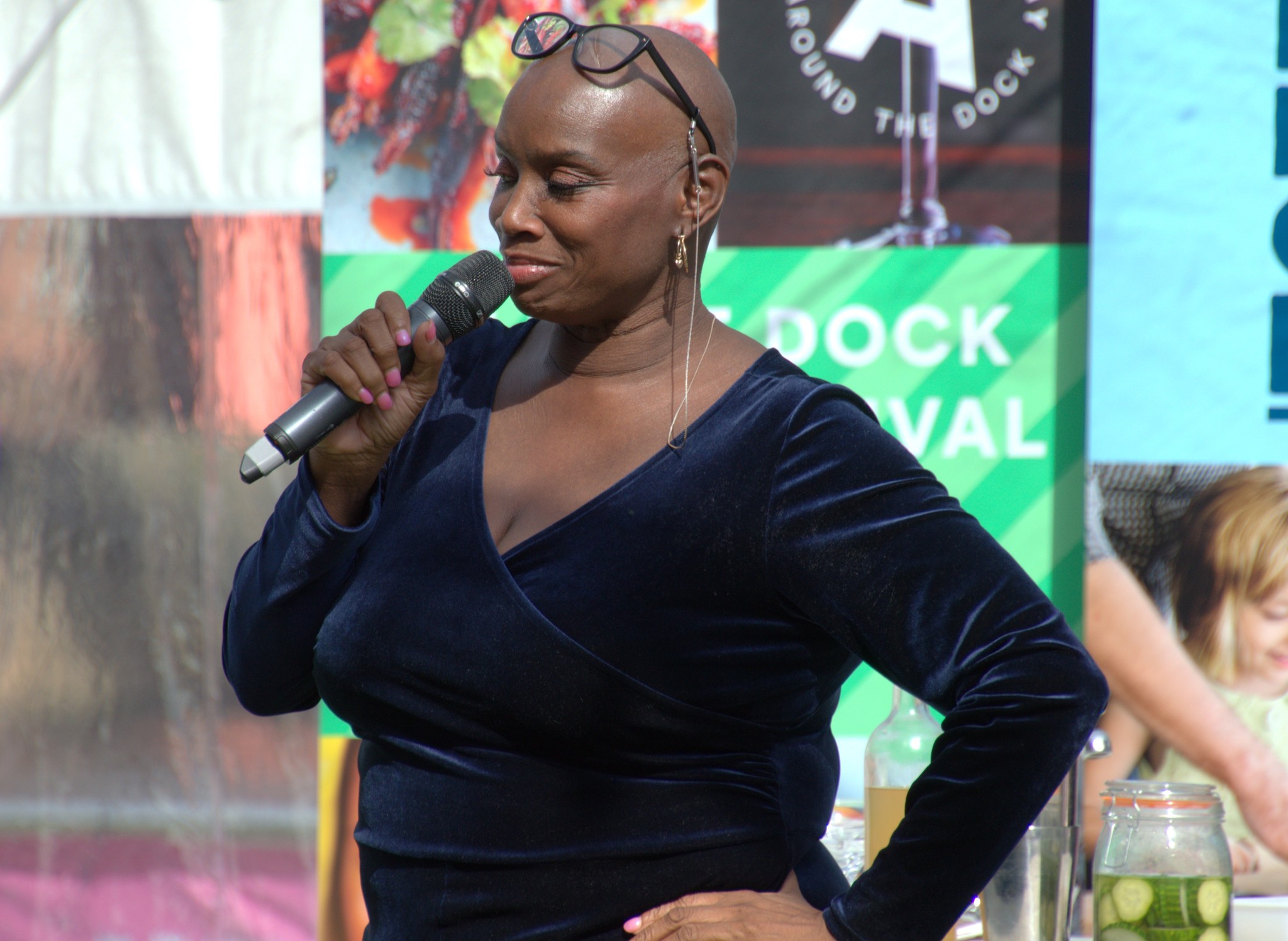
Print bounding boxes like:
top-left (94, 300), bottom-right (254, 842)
top-left (982, 828), bottom-right (1081, 941)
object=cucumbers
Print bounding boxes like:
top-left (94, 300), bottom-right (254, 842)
top-left (1092, 873), bottom-right (1231, 941)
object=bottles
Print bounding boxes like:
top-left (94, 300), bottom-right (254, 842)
top-left (863, 677), bottom-right (959, 941)
top-left (1091, 780), bottom-right (1234, 941)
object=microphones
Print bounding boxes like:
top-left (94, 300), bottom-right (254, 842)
top-left (239, 249), bottom-right (515, 484)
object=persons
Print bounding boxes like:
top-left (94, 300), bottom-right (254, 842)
top-left (220, 22), bottom-right (1108, 941)
top-left (1083, 461), bottom-right (1288, 898)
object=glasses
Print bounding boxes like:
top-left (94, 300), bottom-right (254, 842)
top-left (511, 11), bottom-right (717, 155)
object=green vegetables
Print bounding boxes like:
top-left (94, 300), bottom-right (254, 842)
top-left (359, 0), bottom-right (639, 130)
